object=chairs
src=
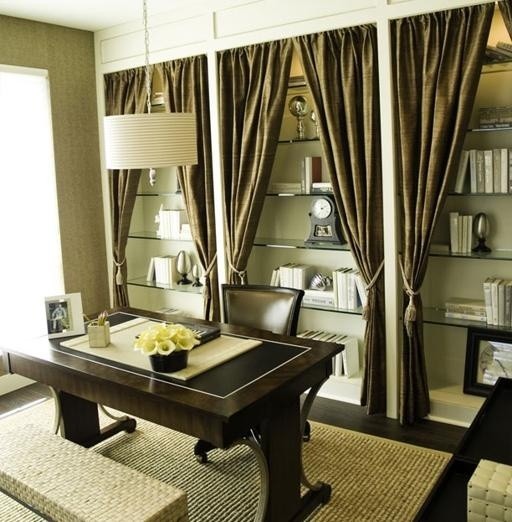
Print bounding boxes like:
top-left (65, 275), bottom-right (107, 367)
top-left (194, 286), bottom-right (313, 463)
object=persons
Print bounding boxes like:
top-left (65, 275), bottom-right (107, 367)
top-left (52, 304), bottom-right (66, 331)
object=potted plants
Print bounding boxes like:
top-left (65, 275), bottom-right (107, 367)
top-left (88, 309), bottom-right (111, 349)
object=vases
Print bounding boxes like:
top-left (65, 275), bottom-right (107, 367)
top-left (150, 350), bottom-right (190, 373)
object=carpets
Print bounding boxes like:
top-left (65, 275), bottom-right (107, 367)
top-left (2, 387), bottom-right (451, 522)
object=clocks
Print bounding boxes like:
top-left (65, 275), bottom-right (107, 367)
top-left (305, 195), bottom-right (344, 245)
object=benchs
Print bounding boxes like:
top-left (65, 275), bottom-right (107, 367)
top-left (1, 422), bottom-right (187, 522)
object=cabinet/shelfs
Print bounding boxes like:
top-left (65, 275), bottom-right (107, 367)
top-left (92, 1), bottom-right (511, 429)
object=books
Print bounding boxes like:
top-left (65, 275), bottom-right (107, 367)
top-left (147, 255), bottom-right (181, 285)
top-left (445, 277), bottom-right (512, 326)
top-left (160, 209), bottom-right (192, 238)
top-left (454, 148), bottom-right (512, 193)
top-left (296, 330), bottom-right (359, 378)
top-left (270, 263), bottom-right (368, 311)
top-left (272, 156), bottom-right (333, 193)
top-left (429, 212), bottom-right (477, 253)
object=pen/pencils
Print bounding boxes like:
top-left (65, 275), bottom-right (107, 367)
top-left (97, 311), bottom-right (108, 327)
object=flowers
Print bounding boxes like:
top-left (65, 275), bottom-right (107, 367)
top-left (133, 319), bottom-right (200, 357)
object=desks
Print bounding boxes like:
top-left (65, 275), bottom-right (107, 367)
top-left (4, 305), bottom-right (344, 522)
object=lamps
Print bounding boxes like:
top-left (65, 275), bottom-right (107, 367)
top-left (99, 0), bottom-right (199, 187)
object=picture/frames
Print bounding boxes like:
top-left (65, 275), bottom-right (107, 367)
top-left (463, 329), bottom-right (511, 397)
top-left (44, 292), bottom-right (90, 342)
